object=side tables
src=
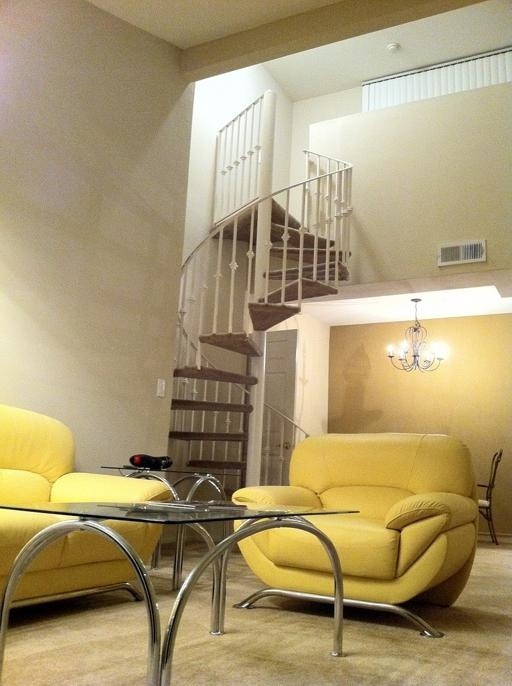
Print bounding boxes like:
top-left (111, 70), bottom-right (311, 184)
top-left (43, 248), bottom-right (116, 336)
top-left (101, 466), bottom-right (243, 592)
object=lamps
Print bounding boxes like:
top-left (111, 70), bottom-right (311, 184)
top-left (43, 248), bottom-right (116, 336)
top-left (386, 298), bottom-right (448, 374)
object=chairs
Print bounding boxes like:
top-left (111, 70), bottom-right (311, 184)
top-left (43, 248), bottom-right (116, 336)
top-left (475, 448), bottom-right (504, 544)
top-left (0, 404), bottom-right (170, 613)
top-left (232, 432), bottom-right (478, 641)
